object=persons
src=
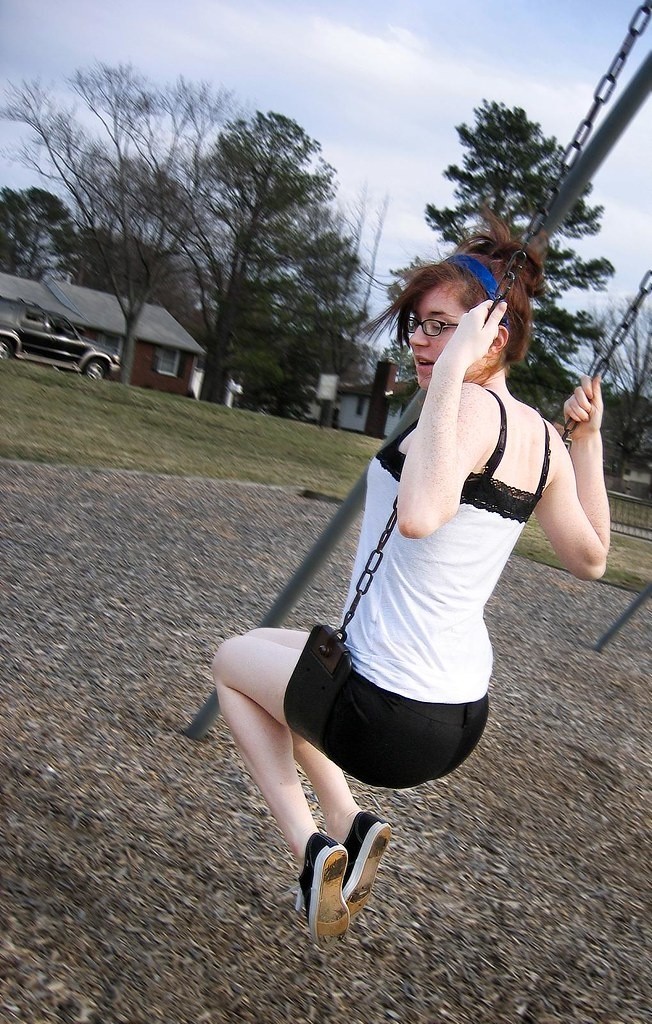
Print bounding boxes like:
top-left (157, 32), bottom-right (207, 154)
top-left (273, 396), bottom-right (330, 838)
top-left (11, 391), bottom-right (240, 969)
top-left (209, 205), bottom-right (611, 940)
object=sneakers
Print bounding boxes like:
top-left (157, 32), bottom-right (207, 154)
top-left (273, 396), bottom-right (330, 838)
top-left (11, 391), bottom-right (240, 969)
top-left (341, 811), bottom-right (391, 920)
top-left (298, 832), bottom-right (350, 952)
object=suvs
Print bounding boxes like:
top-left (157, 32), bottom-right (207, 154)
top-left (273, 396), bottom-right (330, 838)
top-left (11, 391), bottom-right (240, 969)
top-left (0, 297), bottom-right (121, 381)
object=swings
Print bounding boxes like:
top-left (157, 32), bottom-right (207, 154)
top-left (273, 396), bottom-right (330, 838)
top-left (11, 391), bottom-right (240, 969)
top-left (281, 0), bottom-right (652, 761)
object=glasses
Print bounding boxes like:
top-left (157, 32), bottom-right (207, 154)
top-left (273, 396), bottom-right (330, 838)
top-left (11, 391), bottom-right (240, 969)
top-left (408, 317), bottom-right (459, 336)
